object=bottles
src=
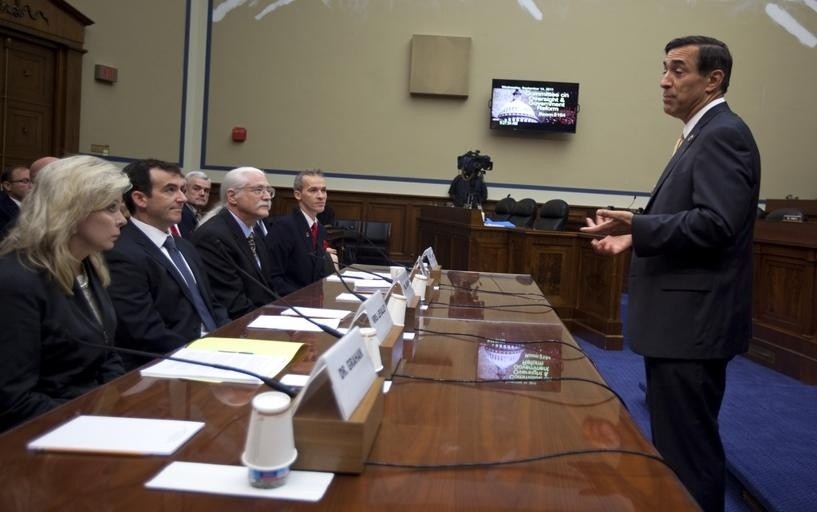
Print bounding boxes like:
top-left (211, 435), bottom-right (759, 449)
top-left (421, 263), bottom-right (430, 277)
top-left (248, 465), bottom-right (289, 488)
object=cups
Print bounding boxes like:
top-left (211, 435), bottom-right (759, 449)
top-left (386, 294), bottom-right (407, 327)
top-left (411, 274), bottom-right (427, 300)
top-left (389, 267), bottom-right (405, 278)
top-left (240, 390), bottom-right (298, 469)
top-left (358, 327), bottom-right (385, 373)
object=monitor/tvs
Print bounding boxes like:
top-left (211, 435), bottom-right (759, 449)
top-left (489, 78), bottom-right (581, 135)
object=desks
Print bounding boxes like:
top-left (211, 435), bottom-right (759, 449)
top-left (0, 262), bottom-right (697, 512)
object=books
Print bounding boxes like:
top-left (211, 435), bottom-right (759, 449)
top-left (245, 310), bottom-right (342, 335)
top-left (144, 456), bottom-right (336, 504)
top-left (28, 409), bottom-right (209, 463)
top-left (324, 266), bottom-right (399, 303)
top-left (138, 331), bottom-right (310, 390)
top-left (279, 299), bottom-right (354, 320)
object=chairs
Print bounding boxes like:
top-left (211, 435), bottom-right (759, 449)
top-left (360, 221), bottom-right (392, 260)
top-left (333, 220), bottom-right (360, 261)
top-left (532, 200), bottom-right (569, 230)
top-left (506, 198), bottom-right (536, 228)
top-left (491, 197), bottom-right (514, 222)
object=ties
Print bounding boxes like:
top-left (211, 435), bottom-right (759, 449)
top-left (246, 231), bottom-right (257, 260)
top-left (671, 132), bottom-right (685, 157)
top-left (170, 223), bottom-right (180, 238)
top-left (162, 234), bottom-right (217, 333)
top-left (311, 222), bottom-right (319, 251)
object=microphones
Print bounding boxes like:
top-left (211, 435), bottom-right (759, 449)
top-left (61, 326), bottom-right (297, 401)
top-left (312, 239), bottom-right (367, 301)
top-left (347, 224), bottom-right (411, 270)
top-left (304, 250), bottom-right (392, 284)
top-left (623, 194), bottom-right (637, 215)
top-left (214, 236), bottom-right (343, 340)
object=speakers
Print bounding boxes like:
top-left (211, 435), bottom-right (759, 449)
top-left (408, 34), bottom-right (474, 98)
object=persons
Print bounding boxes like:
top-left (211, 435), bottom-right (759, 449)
top-left (167, 173), bottom-right (192, 242)
top-left (575, 33), bottom-right (762, 509)
top-left (0, 154), bottom-right (127, 441)
top-left (187, 162), bottom-right (305, 330)
top-left (99, 155), bottom-right (234, 372)
top-left (29, 155), bottom-right (60, 188)
top-left (0, 163), bottom-right (35, 245)
top-left (178, 171), bottom-right (213, 244)
top-left (258, 169), bottom-right (341, 301)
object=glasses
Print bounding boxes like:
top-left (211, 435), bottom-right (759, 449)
top-left (9, 177), bottom-right (32, 185)
top-left (241, 184), bottom-right (277, 199)
